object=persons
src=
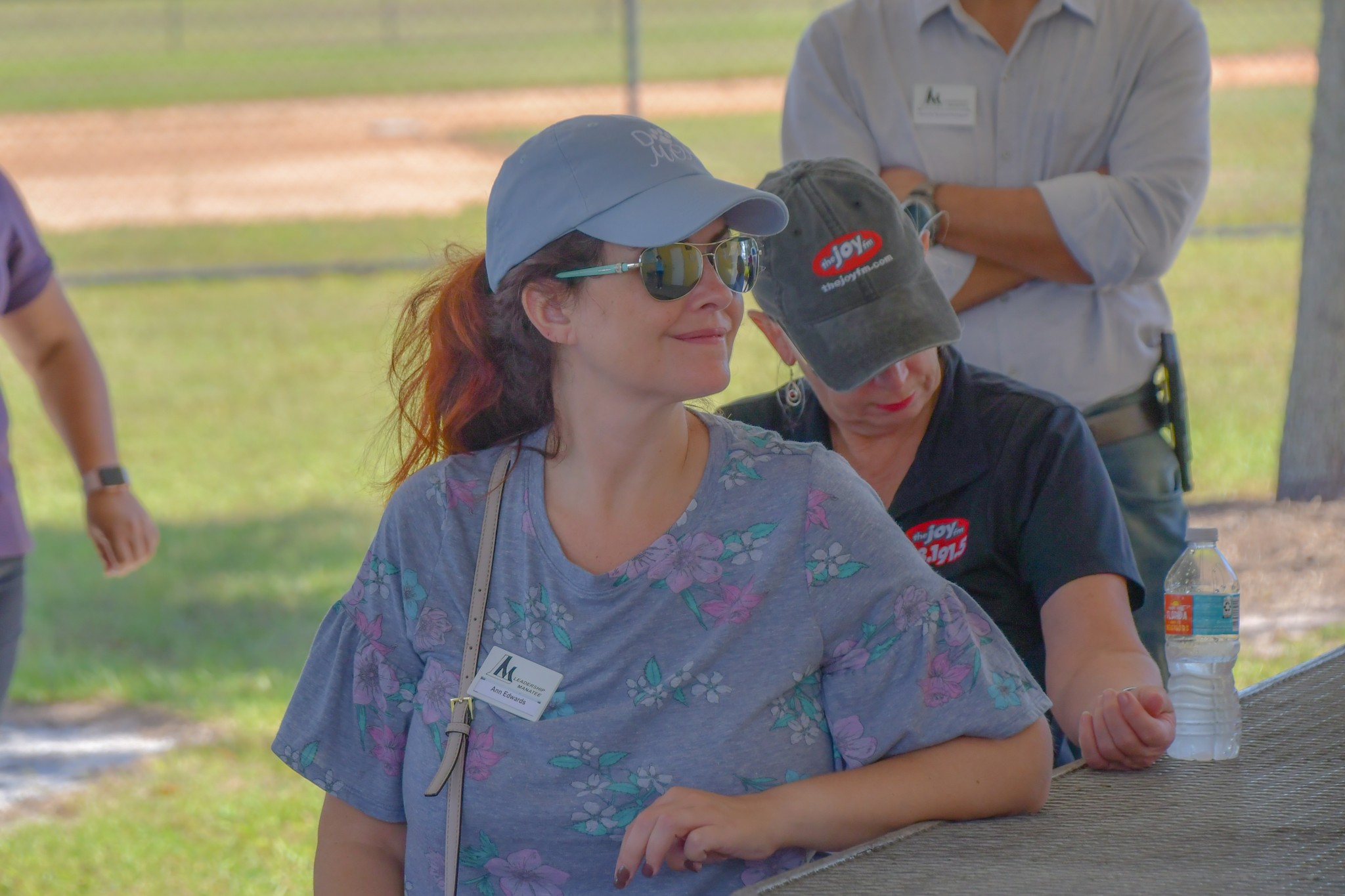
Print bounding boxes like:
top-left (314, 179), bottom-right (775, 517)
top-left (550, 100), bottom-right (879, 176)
top-left (779, 1), bottom-right (1211, 693)
top-left (270, 112), bottom-right (1054, 895)
top-left (0, 174), bottom-right (158, 703)
top-left (710, 158), bottom-right (1176, 770)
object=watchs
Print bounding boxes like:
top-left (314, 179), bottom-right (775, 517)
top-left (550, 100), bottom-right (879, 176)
top-left (84, 466), bottom-right (131, 495)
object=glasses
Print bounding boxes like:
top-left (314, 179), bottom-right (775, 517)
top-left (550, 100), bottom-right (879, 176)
top-left (556, 235), bottom-right (763, 300)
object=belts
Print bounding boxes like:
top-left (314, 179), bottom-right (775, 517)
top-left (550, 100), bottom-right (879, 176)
top-left (1088, 392), bottom-right (1166, 447)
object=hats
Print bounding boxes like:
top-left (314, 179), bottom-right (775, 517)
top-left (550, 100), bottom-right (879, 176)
top-left (737, 154), bottom-right (963, 391)
top-left (484, 113), bottom-right (789, 294)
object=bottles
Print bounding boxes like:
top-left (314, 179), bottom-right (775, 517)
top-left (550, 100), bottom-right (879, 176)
top-left (1161, 526), bottom-right (1247, 760)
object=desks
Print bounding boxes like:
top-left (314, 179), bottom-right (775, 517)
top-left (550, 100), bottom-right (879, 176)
top-left (732, 643), bottom-right (1342, 894)
